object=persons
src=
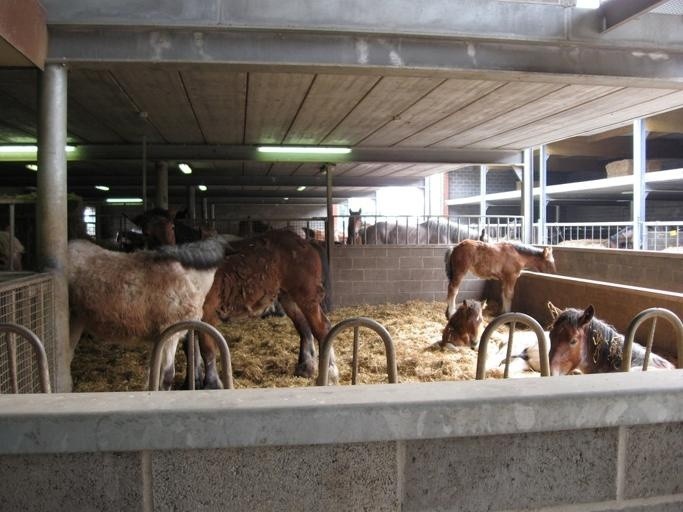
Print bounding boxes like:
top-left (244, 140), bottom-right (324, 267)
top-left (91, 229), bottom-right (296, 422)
top-left (0, 217), bottom-right (27, 272)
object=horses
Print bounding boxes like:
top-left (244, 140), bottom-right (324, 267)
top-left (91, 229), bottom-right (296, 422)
top-left (168, 207), bottom-right (334, 248)
top-left (445, 239), bottom-right (556, 321)
top-left (557, 226), bottom-right (683, 253)
top-left (66, 228), bottom-right (238, 393)
top-left (442, 297), bottom-right (677, 378)
top-left (0, 224), bottom-right (26, 271)
top-left (119, 207), bottom-right (339, 389)
top-left (345, 209), bottom-right (517, 244)
